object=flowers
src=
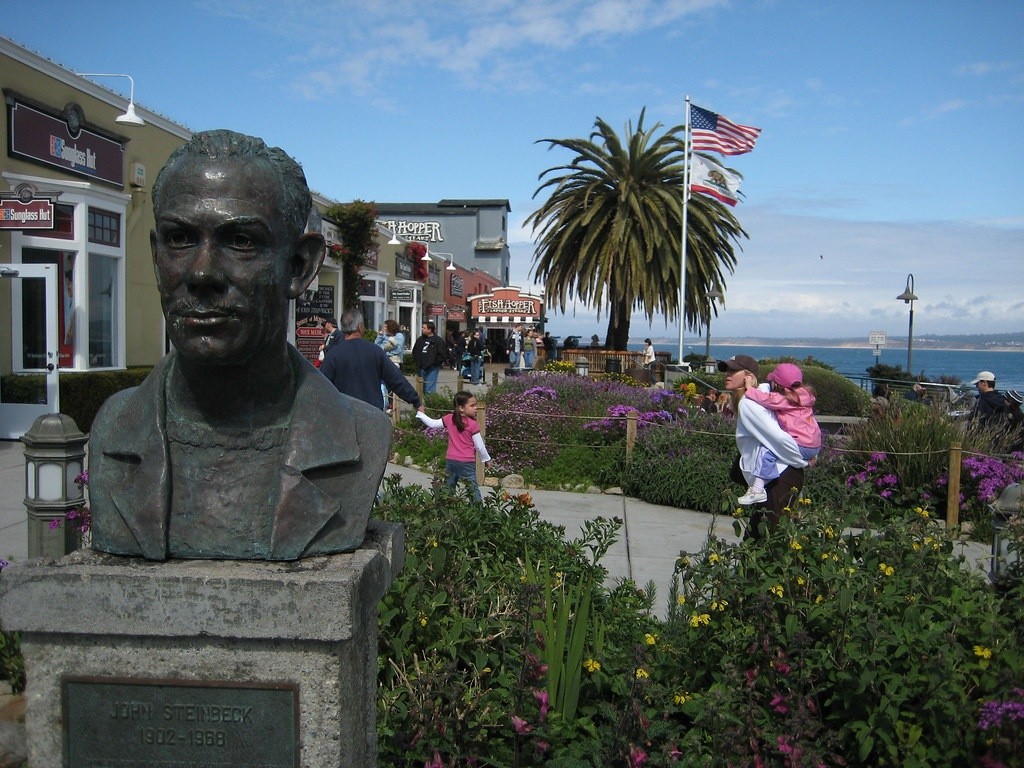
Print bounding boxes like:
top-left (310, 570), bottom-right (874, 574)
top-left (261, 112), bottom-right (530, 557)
top-left (404, 242), bottom-right (428, 282)
top-left (327, 195), bottom-right (379, 309)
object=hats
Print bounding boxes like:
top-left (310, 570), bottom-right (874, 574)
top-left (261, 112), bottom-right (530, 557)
top-left (321, 318), bottom-right (337, 327)
top-left (767, 363), bottom-right (803, 390)
top-left (1002, 390), bottom-right (1024, 405)
top-left (970, 371), bottom-right (996, 384)
top-left (717, 354), bottom-right (758, 381)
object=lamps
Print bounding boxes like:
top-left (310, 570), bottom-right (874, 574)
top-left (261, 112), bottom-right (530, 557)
top-left (406, 240), bottom-right (432, 261)
top-left (132, 188), bottom-right (147, 209)
top-left (705, 356), bottom-right (717, 374)
top-left (19, 414), bottom-right (90, 510)
top-left (430, 252), bottom-right (456, 270)
top-left (76, 73), bottom-right (146, 127)
top-left (992, 485), bottom-right (1024, 574)
top-left (575, 357), bottom-right (589, 375)
top-left (384, 221), bottom-right (400, 244)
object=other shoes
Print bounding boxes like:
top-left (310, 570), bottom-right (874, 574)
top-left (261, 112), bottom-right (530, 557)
top-left (386, 408), bottom-right (392, 414)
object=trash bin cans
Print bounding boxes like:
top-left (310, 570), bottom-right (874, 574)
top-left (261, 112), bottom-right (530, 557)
top-left (605, 358), bottom-right (621, 373)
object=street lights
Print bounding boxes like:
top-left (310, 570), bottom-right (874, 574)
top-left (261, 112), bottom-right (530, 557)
top-left (701, 272), bottom-right (722, 357)
top-left (896, 273), bottom-right (919, 374)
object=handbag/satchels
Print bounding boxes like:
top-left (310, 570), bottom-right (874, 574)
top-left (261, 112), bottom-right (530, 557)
top-left (730, 453), bottom-right (747, 487)
top-left (319, 350), bottom-right (325, 362)
top-left (642, 356), bottom-right (648, 364)
top-left (519, 355), bottom-right (526, 368)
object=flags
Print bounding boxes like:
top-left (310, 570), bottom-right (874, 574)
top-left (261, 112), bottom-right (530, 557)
top-left (689, 150), bottom-right (743, 208)
top-left (690, 103), bottom-right (761, 156)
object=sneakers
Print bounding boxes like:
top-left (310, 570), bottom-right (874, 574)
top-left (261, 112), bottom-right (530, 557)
top-left (737, 486), bottom-right (767, 506)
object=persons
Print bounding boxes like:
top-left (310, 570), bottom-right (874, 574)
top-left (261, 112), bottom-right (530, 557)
top-left (414, 391), bottom-right (494, 508)
top-left (87, 129), bottom-right (393, 562)
top-left (507, 324), bottom-right (556, 369)
top-left (716, 354), bottom-right (818, 547)
top-left (446, 326), bottom-right (487, 386)
top-left (970, 371), bottom-right (1007, 453)
top-left (319, 309), bottom-right (426, 414)
top-left (642, 339), bottom-right (656, 369)
top-left (591, 334), bottom-right (600, 346)
top-left (412, 321), bottom-right (450, 399)
top-left (1001, 391), bottom-right (1024, 455)
top-left (374, 320), bottom-right (405, 416)
top-left (64, 254), bottom-right (75, 345)
top-left (404, 323), bottom-right (411, 345)
top-left (737, 363), bottom-right (822, 506)
top-left (319, 318), bottom-right (345, 354)
